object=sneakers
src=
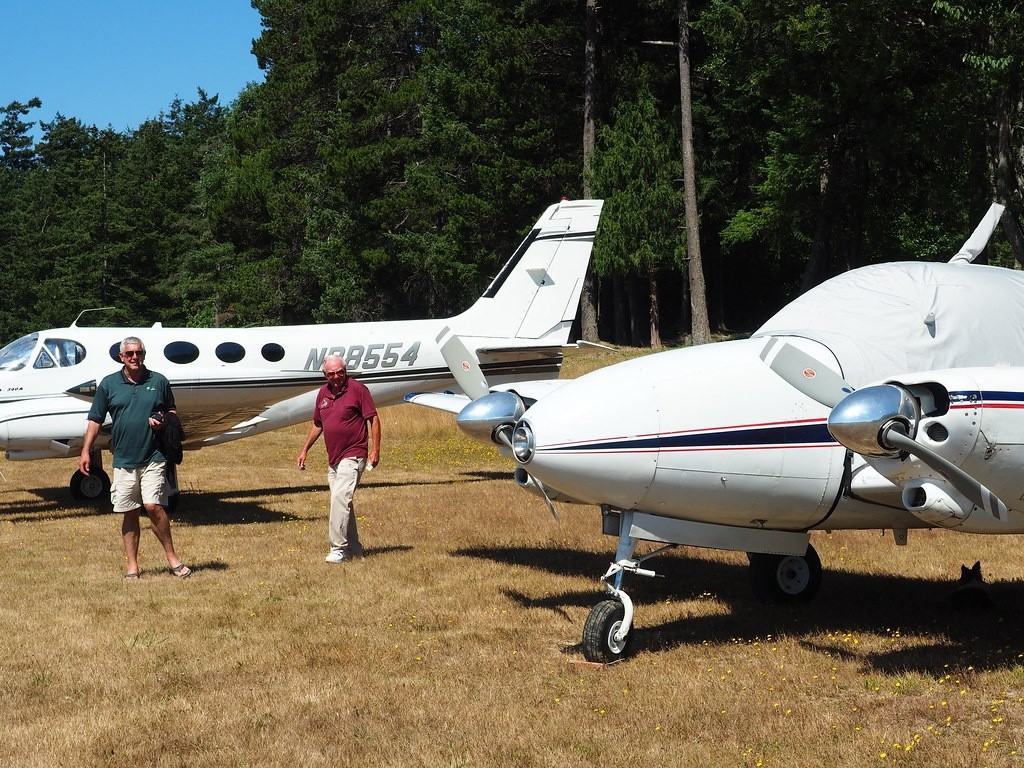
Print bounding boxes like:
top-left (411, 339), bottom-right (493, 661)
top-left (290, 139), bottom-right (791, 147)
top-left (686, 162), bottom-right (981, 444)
top-left (326, 549), bottom-right (352, 564)
top-left (343, 547), bottom-right (363, 556)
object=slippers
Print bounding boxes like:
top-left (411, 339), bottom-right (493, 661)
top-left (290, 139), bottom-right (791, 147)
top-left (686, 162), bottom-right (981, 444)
top-left (125, 573), bottom-right (140, 580)
top-left (171, 563), bottom-right (191, 578)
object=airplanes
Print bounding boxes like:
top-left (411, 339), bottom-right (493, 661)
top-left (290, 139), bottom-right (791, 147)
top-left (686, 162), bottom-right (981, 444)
top-left (0, 198), bottom-right (620, 508)
top-left (404, 202), bottom-right (1024, 663)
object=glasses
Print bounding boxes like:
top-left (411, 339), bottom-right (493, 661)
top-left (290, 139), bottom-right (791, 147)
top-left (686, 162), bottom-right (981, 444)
top-left (325, 369), bottom-right (345, 379)
top-left (121, 350), bottom-right (143, 357)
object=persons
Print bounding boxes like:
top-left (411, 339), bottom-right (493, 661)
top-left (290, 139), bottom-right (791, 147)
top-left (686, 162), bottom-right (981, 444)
top-left (297, 356), bottom-right (381, 563)
top-left (79, 337), bottom-right (191, 579)
